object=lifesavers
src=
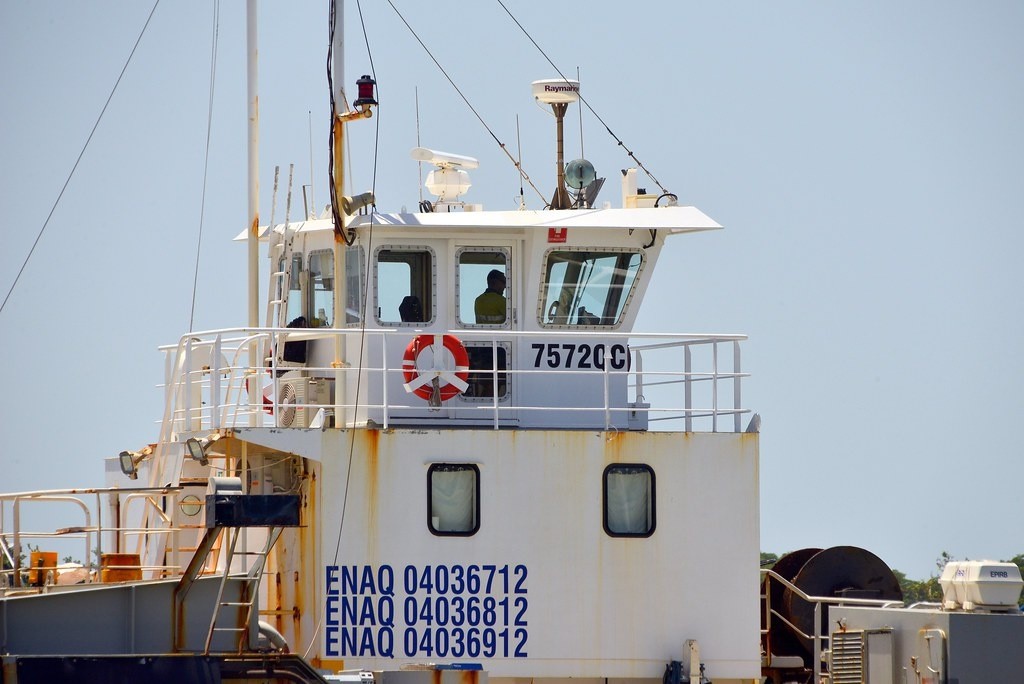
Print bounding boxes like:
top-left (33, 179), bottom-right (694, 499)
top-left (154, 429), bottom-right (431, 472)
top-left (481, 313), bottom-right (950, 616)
top-left (402, 334), bottom-right (470, 401)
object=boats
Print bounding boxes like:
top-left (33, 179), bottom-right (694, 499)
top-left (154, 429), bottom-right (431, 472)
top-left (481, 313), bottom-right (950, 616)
top-left (0, 2), bottom-right (1024, 683)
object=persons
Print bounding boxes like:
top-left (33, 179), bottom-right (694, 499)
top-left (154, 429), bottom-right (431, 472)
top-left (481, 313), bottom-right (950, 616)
top-left (474, 269), bottom-right (507, 324)
